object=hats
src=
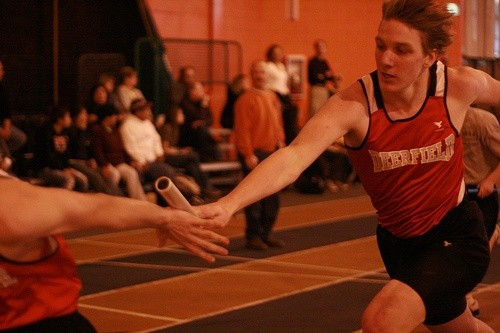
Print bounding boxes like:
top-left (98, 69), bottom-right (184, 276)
top-left (131, 97), bottom-right (152, 112)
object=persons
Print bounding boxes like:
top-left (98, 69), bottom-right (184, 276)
top-left (462, 105), bottom-right (500, 315)
top-left (0, 40), bottom-right (358, 249)
top-left (188, 0), bottom-right (500, 333)
top-left (0, 170), bottom-right (230, 333)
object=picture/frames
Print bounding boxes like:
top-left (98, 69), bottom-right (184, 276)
top-left (286, 54), bottom-right (307, 98)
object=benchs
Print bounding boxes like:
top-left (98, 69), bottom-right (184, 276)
top-left (28, 161), bottom-right (242, 192)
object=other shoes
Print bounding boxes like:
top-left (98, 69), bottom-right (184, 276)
top-left (331, 179), bottom-right (349, 190)
top-left (264, 231), bottom-right (285, 246)
top-left (246, 237), bottom-right (269, 248)
top-left (324, 181), bottom-right (338, 191)
